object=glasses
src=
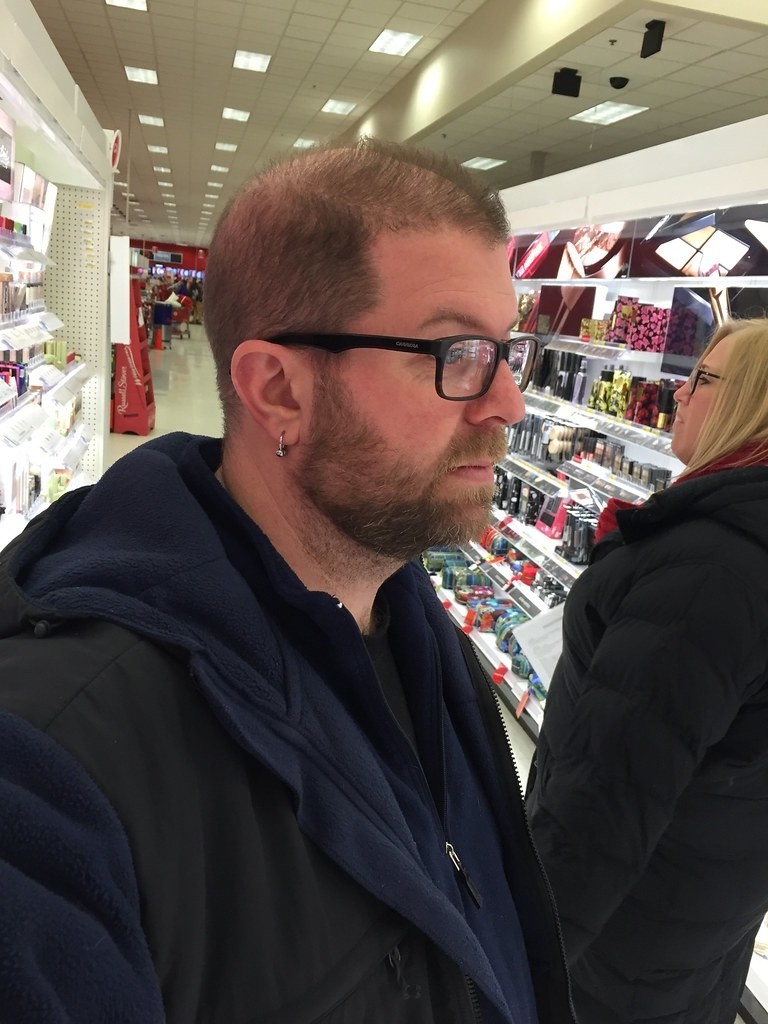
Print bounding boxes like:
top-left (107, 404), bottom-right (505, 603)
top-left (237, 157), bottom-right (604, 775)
top-left (690, 370), bottom-right (735, 396)
top-left (265, 334), bottom-right (541, 401)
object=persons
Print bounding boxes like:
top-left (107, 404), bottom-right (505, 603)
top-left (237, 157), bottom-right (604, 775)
top-left (0, 139), bottom-right (575, 1023)
top-left (171, 273), bottom-right (205, 325)
top-left (525, 320), bottom-right (768, 1023)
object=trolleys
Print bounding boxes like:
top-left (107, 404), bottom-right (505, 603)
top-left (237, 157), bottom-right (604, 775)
top-left (171, 306), bottom-right (191, 340)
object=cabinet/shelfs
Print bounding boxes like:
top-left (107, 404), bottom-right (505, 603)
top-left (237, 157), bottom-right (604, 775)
top-left (419, 192), bottom-right (767, 747)
top-left (0, 0), bottom-right (113, 552)
